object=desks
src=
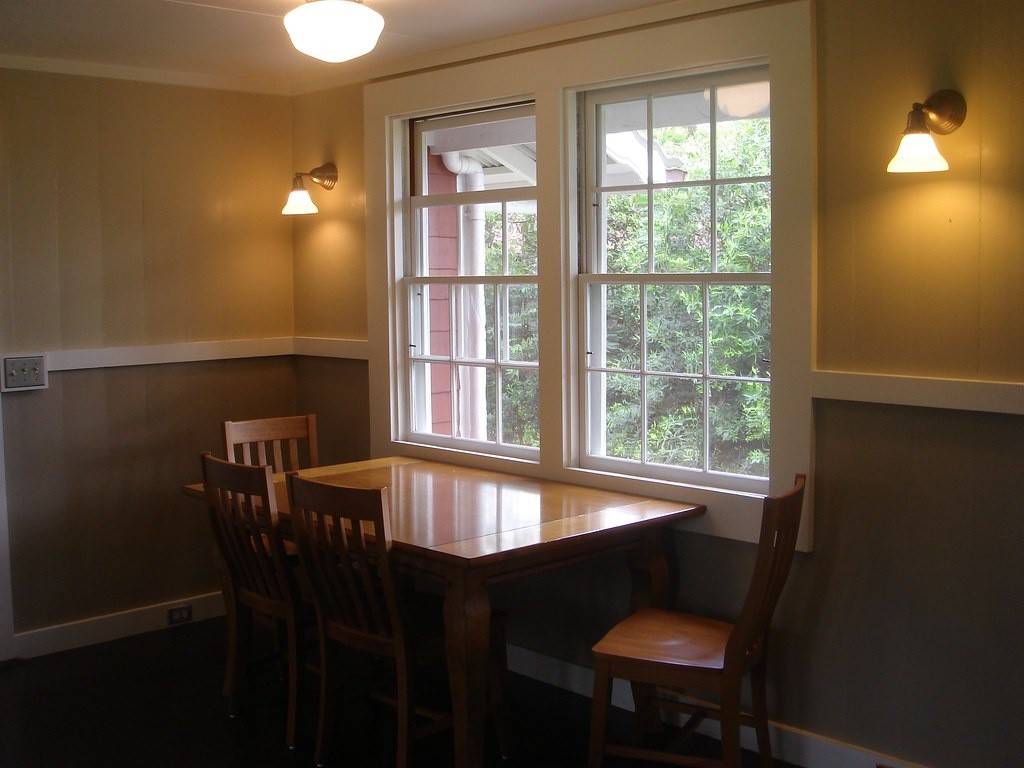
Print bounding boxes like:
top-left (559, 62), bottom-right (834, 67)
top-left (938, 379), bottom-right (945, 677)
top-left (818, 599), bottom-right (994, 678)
top-left (181, 455), bottom-right (707, 768)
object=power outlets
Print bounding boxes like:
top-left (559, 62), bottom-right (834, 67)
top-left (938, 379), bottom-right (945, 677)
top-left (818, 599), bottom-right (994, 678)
top-left (167, 605), bottom-right (193, 625)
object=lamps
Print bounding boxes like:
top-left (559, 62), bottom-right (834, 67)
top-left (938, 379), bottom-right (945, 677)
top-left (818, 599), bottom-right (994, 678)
top-left (886, 89), bottom-right (967, 172)
top-left (283, 0), bottom-right (385, 64)
top-left (281, 162), bottom-right (338, 215)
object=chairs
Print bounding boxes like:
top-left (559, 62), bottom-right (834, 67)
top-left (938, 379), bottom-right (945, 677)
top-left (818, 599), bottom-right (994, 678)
top-left (200, 450), bottom-right (362, 748)
top-left (284, 470), bottom-right (511, 768)
top-left (589, 472), bottom-right (807, 768)
top-left (220, 413), bottom-right (320, 566)
